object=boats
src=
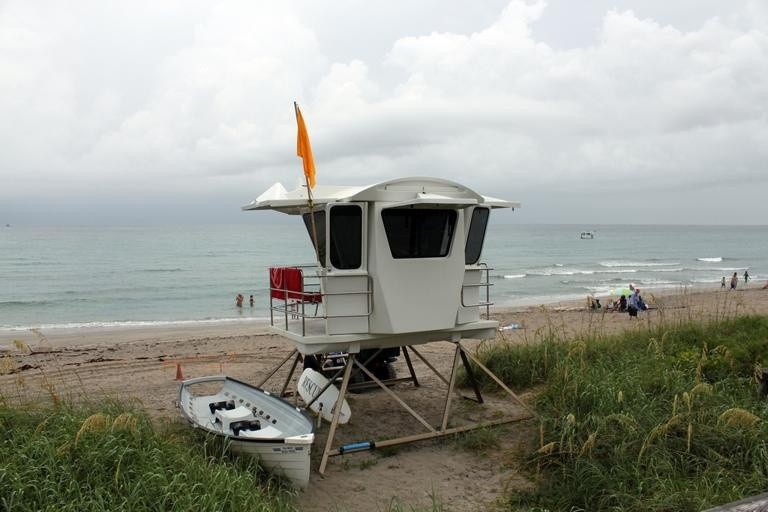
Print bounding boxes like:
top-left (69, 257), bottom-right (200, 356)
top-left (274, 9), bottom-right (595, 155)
top-left (174, 374), bottom-right (316, 492)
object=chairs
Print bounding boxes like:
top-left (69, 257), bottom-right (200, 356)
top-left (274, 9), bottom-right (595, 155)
top-left (269, 266), bottom-right (326, 317)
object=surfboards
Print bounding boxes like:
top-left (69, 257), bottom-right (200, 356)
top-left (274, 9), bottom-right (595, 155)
top-left (298, 368), bottom-right (350, 427)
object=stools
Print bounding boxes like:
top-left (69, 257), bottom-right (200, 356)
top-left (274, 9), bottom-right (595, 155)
top-left (212, 405), bottom-right (284, 441)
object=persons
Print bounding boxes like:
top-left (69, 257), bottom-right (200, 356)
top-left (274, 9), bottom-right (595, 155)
top-left (585, 283), bottom-right (648, 320)
top-left (248, 295), bottom-right (254, 307)
top-left (287, 300), bottom-right (297, 320)
top-left (235, 294), bottom-right (244, 308)
top-left (720, 275), bottom-right (725, 287)
top-left (730, 271), bottom-right (738, 289)
top-left (743, 271), bottom-right (750, 283)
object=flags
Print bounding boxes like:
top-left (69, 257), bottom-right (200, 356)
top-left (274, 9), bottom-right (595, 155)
top-left (294, 105), bottom-right (318, 192)
